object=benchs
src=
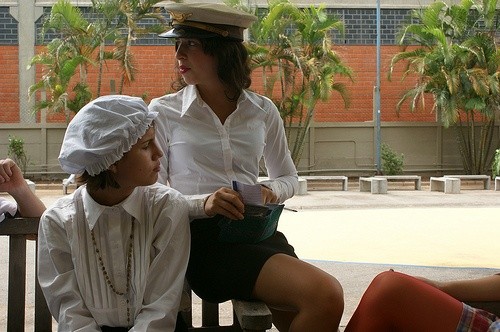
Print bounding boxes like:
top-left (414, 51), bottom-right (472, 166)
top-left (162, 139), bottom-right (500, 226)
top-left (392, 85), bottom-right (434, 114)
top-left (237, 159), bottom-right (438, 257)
top-left (359, 177), bottom-right (387, 194)
top-left (305, 176), bottom-right (348, 190)
top-left (1, 214), bottom-right (273, 332)
top-left (374, 175), bottom-right (422, 190)
top-left (444, 174), bottom-right (490, 190)
top-left (430, 178), bottom-right (460, 194)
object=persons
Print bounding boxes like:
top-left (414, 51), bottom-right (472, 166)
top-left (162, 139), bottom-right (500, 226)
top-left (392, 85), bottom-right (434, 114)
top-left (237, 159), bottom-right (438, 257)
top-left (344, 269), bottom-right (500, 332)
top-left (148, 0), bottom-right (344, 332)
top-left (0, 159), bottom-right (47, 240)
top-left (38, 94), bottom-right (192, 332)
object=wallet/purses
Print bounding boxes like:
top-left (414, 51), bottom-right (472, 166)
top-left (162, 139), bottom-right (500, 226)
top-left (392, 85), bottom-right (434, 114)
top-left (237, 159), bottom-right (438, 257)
top-left (216, 204), bottom-right (285, 242)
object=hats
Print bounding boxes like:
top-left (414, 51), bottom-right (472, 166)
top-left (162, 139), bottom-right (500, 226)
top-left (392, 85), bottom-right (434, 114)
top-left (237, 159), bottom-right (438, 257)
top-left (58, 95), bottom-right (160, 178)
top-left (158, 3), bottom-right (258, 42)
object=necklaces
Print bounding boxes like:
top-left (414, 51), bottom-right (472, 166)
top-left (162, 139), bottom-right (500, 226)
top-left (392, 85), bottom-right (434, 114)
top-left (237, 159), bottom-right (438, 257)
top-left (89, 216), bottom-right (137, 329)
